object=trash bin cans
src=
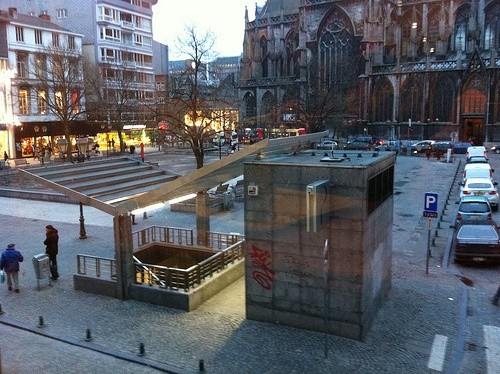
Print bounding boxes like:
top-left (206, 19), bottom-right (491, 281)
top-left (32, 252), bottom-right (53, 291)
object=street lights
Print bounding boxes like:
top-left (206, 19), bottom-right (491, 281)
top-left (69, 150), bottom-right (87, 239)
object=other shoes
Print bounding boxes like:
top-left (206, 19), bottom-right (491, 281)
top-left (8, 287), bottom-right (12, 291)
top-left (15, 290), bottom-right (19, 293)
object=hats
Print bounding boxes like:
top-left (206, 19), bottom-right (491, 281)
top-left (7, 243), bottom-right (15, 248)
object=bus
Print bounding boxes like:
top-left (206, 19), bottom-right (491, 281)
top-left (250, 127), bottom-right (307, 143)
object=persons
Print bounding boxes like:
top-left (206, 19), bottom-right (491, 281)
top-left (426, 143), bottom-right (432, 159)
top-left (436, 145), bottom-right (441, 160)
top-left (4, 151), bottom-right (10, 165)
top-left (44, 225), bottom-right (60, 281)
top-left (374, 144), bottom-right (380, 152)
top-left (107, 138), bottom-right (115, 148)
top-left (385, 146), bottom-right (391, 151)
top-left (392, 146), bottom-right (398, 163)
top-left (231, 143), bottom-right (237, 154)
top-left (0, 243), bottom-right (24, 293)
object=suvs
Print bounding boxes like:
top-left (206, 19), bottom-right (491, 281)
top-left (465, 146), bottom-right (489, 163)
top-left (461, 163), bottom-right (495, 182)
top-left (458, 178), bottom-right (499, 210)
top-left (454, 195), bottom-right (496, 226)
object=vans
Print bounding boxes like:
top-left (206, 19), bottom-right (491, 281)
top-left (212, 132), bottom-right (239, 145)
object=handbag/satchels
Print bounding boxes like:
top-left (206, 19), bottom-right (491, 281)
top-left (0, 271), bottom-right (6, 283)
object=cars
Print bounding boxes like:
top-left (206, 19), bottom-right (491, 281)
top-left (347, 135), bottom-right (471, 154)
top-left (490, 145), bottom-right (500, 154)
top-left (317, 141), bottom-right (338, 149)
top-left (449, 219), bottom-right (500, 264)
